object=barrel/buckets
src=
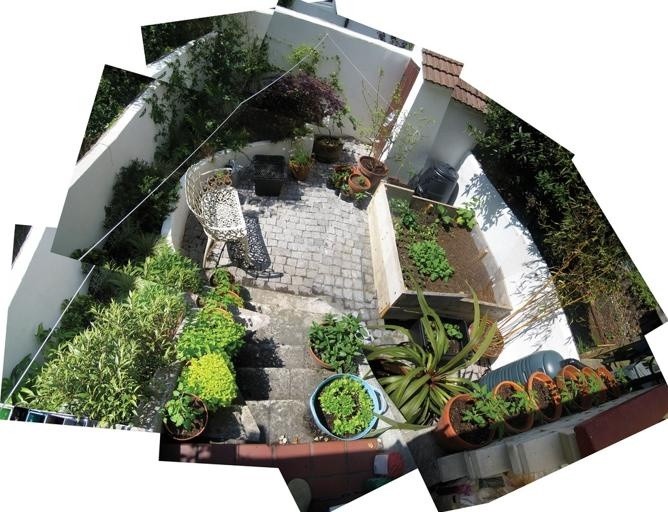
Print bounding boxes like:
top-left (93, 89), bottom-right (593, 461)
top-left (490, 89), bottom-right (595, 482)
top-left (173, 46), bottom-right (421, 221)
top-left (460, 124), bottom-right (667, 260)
top-left (413, 157), bottom-right (460, 204)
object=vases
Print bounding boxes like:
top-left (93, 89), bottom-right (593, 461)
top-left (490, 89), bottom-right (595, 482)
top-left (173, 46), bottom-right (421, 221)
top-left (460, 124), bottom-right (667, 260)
top-left (250, 154), bottom-right (285, 198)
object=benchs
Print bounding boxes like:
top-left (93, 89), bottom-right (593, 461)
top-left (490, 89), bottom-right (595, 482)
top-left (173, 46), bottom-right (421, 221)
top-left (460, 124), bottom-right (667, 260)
top-left (185, 158), bottom-right (249, 270)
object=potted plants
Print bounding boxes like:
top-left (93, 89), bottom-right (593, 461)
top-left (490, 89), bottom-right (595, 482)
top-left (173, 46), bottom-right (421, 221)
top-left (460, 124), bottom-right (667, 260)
top-left (158, 267), bottom-right (628, 453)
top-left (288, 137), bottom-right (314, 181)
top-left (314, 55), bottom-right (437, 207)
top-left (207, 170), bottom-right (232, 187)
